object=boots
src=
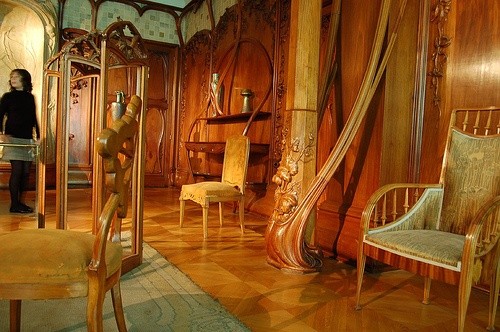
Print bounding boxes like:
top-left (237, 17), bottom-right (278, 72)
top-left (9, 185), bottom-right (33, 213)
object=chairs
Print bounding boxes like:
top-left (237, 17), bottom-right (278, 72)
top-left (179, 134), bottom-right (250, 238)
top-left (355, 106), bottom-right (500, 332)
top-left (0, 95), bottom-right (142, 331)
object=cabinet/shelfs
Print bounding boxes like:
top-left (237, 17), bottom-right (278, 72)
top-left (185, 111), bottom-right (272, 213)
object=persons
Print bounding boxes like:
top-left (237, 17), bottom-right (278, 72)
top-left (0, 68), bottom-right (40, 214)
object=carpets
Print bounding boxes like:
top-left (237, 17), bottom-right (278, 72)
top-left (0, 230), bottom-right (252, 332)
